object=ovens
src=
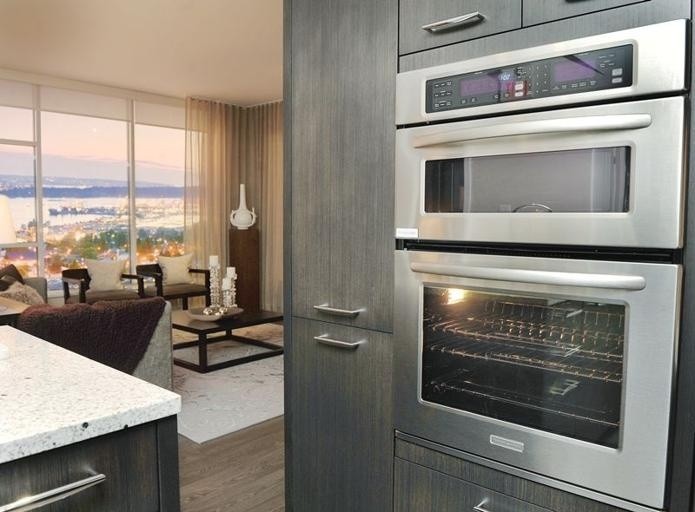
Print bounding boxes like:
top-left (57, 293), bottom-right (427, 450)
top-left (389, 18), bottom-right (687, 512)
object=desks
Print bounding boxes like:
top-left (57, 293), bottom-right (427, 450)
top-left (0, 296), bottom-right (32, 316)
top-left (0, 322), bottom-right (183, 511)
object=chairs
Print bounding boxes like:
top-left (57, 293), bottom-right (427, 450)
top-left (22, 278), bottom-right (47, 304)
top-left (136, 264), bottom-right (210, 310)
top-left (18, 298), bottom-right (173, 391)
top-left (62, 269), bottom-right (145, 303)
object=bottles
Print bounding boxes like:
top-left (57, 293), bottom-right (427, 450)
top-left (203, 307), bottom-right (227, 316)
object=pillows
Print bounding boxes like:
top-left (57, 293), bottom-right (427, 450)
top-left (157, 254), bottom-right (194, 285)
top-left (85, 257), bottom-right (128, 293)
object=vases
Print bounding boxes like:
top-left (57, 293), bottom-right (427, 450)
top-left (229, 184), bottom-right (256, 230)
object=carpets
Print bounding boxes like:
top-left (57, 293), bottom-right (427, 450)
top-left (171, 324), bottom-right (284, 444)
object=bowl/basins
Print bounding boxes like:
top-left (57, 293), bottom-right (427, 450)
top-left (186, 306), bottom-right (245, 322)
